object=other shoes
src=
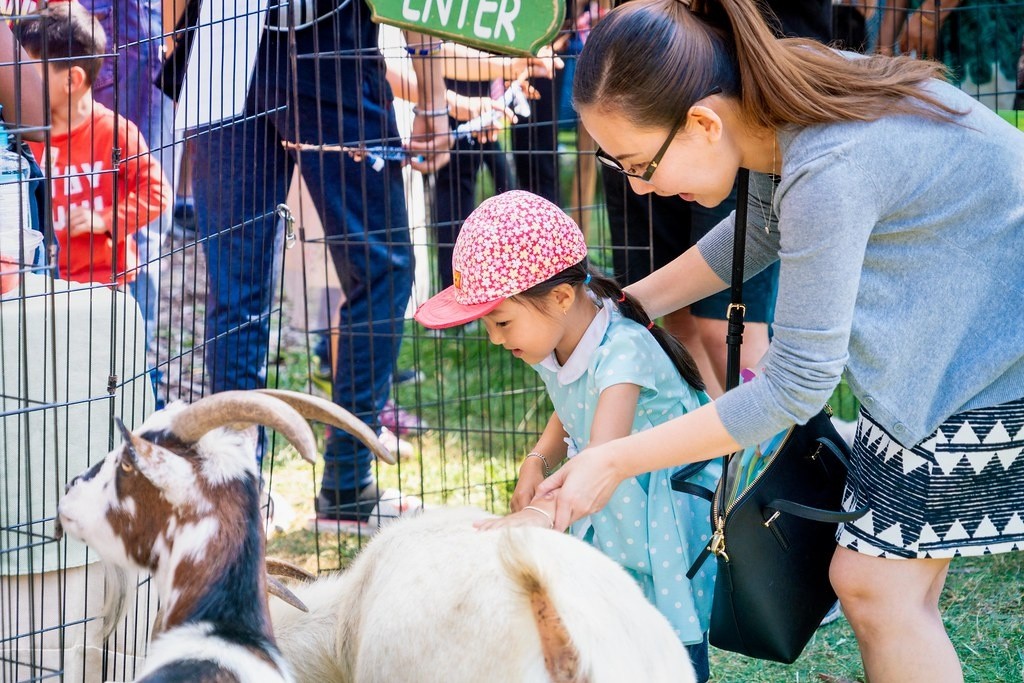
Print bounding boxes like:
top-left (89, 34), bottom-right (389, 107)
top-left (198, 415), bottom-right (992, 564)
top-left (394, 370), bottom-right (425, 387)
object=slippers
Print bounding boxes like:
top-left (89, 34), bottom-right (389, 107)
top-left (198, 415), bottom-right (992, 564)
top-left (308, 487), bottom-right (422, 537)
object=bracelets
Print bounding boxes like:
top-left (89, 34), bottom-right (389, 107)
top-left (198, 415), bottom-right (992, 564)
top-left (407, 39), bottom-right (443, 48)
top-left (525, 453), bottom-right (549, 478)
top-left (523, 506), bottom-right (554, 529)
top-left (413, 103), bottom-right (450, 116)
top-left (405, 45), bottom-right (442, 56)
top-left (915, 12), bottom-right (944, 28)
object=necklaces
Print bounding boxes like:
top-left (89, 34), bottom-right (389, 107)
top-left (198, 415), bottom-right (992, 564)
top-left (751, 129), bottom-right (776, 235)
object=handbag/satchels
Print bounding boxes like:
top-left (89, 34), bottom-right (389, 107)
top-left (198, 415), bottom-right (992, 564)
top-left (670, 403), bottom-right (871, 665)
top-left (154, 0), bottom-right (202, 103)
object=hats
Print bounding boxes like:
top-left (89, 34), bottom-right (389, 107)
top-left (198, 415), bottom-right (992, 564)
top-left (414, 190), bottom-right (588, 329)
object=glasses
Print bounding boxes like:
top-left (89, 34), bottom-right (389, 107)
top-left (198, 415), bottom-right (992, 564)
top-left (595, 85), bottom-right (722, 182)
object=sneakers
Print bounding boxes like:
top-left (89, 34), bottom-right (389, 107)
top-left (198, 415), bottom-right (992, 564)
top-left (168, 197), bottom-right (203, 239)
top-left (378, 400), bottom-right (428, 437)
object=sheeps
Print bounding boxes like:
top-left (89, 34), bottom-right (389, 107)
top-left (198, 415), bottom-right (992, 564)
top-left (60, 388), bottom-right (395, 683)
top-left (262, 506), bottom-right (696, 683)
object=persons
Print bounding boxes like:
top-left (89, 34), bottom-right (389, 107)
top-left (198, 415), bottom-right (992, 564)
top-left (533, 0), bottom-right (1024, 683)
top-left (414, 190), bottom-right (725, 683)
top-left (0, 0), bottom-right (953, 537)
